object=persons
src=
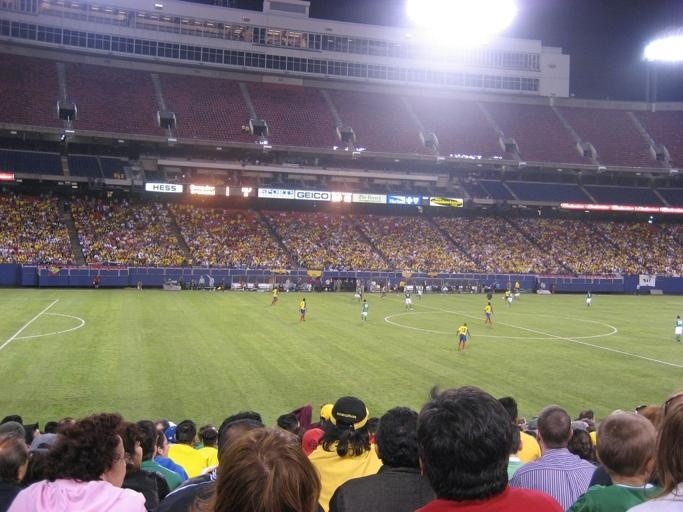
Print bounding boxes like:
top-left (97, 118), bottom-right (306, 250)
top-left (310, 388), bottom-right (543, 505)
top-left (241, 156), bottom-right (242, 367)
top-left (584, 290), bottom-right (592, 307)
top-left (297, 298), bottom-right (306, 321)
top-left (672, 316), bottom-right (682, 342)
top-left (483, 302), bottom-right (495, 324)
top-left (2, 183), bottom-right (681, 292)
top-left (379, 284), bottom-right (387, 299)
top-left (503, 289), bottom-right (510, 307)
top-left (360, 299), bottom-right (369, 320)
top-left (1, 382), bottom-right (683, 512)
top-left (271, 287), bottom-right (277, 305)
top-left (404, 290), bottom-right (412, 311)
top-left (455, 323), bottom-right (471, 351)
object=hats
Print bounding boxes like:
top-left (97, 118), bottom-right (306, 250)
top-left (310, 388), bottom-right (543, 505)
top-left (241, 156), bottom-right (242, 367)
top-left (320, 403), bottom-right (333, 422)
top-left (302, 428), bottom-right (327, 458)
top-left (28, 431), bottom-right (59, 458)
top-left (330, 396), bottom-right (370, 431)
top-left (164, 425), bottom-right (178, 443)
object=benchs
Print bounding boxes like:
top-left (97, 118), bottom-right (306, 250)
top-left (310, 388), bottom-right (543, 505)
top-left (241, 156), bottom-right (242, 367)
top-left (231, 283), bottom-right (311, 291)
top-left (191, 287), bottom-right (216, 291)
top-left (370, 285), bottom-right (477, 292)
